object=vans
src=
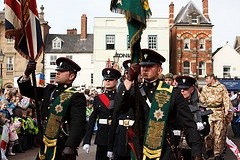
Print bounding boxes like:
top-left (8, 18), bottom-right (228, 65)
top-left (218, 77), bottom-right (240, 93)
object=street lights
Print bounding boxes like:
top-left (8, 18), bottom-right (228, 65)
top-left (112, 49), bottom-right (120, 64)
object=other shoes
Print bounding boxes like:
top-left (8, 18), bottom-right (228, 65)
top-left (8, 152), bottom-right (16, 155)
top-left (215, 156), bottom-right (222, 160)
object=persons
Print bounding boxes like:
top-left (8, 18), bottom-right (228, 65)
top-left (161, 74), bottom-right (240, 160)
top-left (0, 85), bottom-right (41, 160)
top-left (17, 57), bottom-right (87, 160)
top-left (112, 49), bottom-right (201, 160)
top-left (75, 60), bottom-right (144, 160)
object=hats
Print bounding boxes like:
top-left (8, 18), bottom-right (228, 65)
top-left (55, 57), bottom-right (81, 73)
top-left (175, 76), bottom-right (196, 89)
top-left (158, 74), bottom-right (165, 80)
top-left (138, 49), bottom-right (165, 65)
top-left (102, 68), bottom-right (121, 79)
top-left (204, 73), bottom-right (214, 78)
top-left (123, 60), bottom-right (131, 73)
top-left (165, 73), bottom-right (174, 79)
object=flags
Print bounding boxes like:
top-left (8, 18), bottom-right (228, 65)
top-left (3, 0), bottom-right (44, 61)
top-left (110, 0), bottom-right (152, 63)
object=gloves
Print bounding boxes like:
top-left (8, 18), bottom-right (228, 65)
top-left (25, 61), bottom-right (38, 76)
top-left (196, 122), bottom-right (204, 131)
top-left (107, 151), bottom-right (114, 160)
top-left (83, 144), bottom-right (90, 154)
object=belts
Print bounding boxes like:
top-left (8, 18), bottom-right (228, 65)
top-left (173, 130), bottom-right (181, 136)
top-left (118, 119), bottom-right (135, 126)
top-left (99, 118), bottom-right (112, 125)
